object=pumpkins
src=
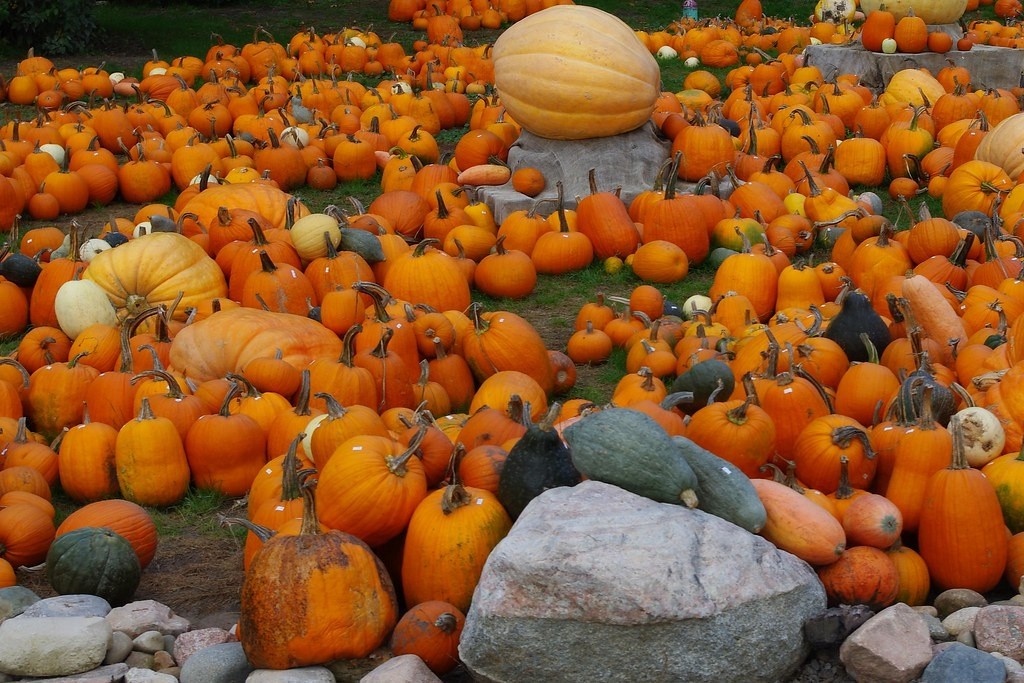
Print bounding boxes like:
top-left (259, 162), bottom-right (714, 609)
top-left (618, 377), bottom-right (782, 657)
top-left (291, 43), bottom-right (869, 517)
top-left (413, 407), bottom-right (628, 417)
top-left (0, 0), bottom-right (1024, 672)
